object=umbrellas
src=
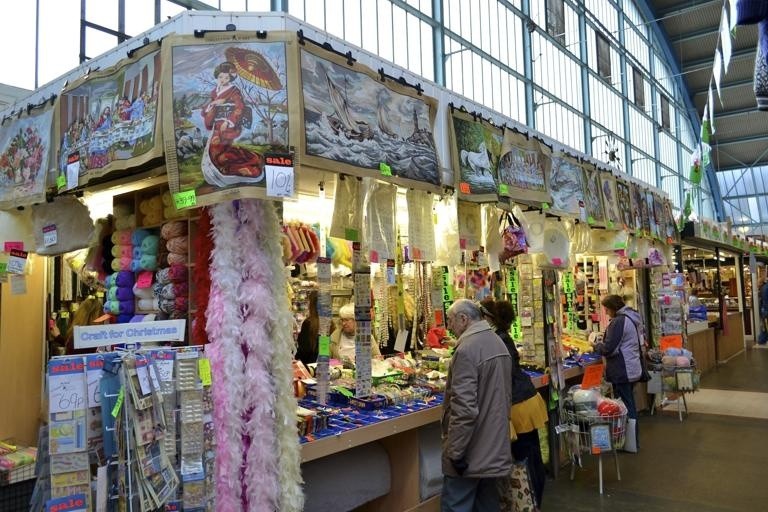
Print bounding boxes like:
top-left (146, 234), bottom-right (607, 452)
top-left (222, 47), bottom-right (283, 105)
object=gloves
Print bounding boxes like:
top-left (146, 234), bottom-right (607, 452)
top-left (442, 454), bottom-right (469, 478)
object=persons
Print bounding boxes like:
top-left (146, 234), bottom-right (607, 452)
top-left (370, 289), bottom-right (399, 355)
top-left (60, 82), bottom-right (157, 166)
top-left (440, 298), bottom-right (513, 512)
top-left (758, 283), bottom-right (768, 344)
top-left (294, 289), bottom-right (335, 364)
top-left (474, 298), bottom-right (550, 509)
top-left (589, 294), bottom-right (645, 454)
top-left (201, 62), bottom-right (265, 189)
top-left (405, 313), bottom-right (425, 350)
top-left (330, 304), bottom-right (381, 366)
top-left (688, 296), bottom-right (707, 321)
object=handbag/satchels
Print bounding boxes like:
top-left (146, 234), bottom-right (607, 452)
top-left (495, 464), bottom-right (537, 512)
top-left (639, 369), bottom-right (651, 383)
top-left (624, 419), bottom-right (637, 453)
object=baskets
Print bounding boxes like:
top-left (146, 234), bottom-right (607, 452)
top-left (563, 409), bottom-right (629, 456)
top-left (648, 357), bottom-right (698, 392)
